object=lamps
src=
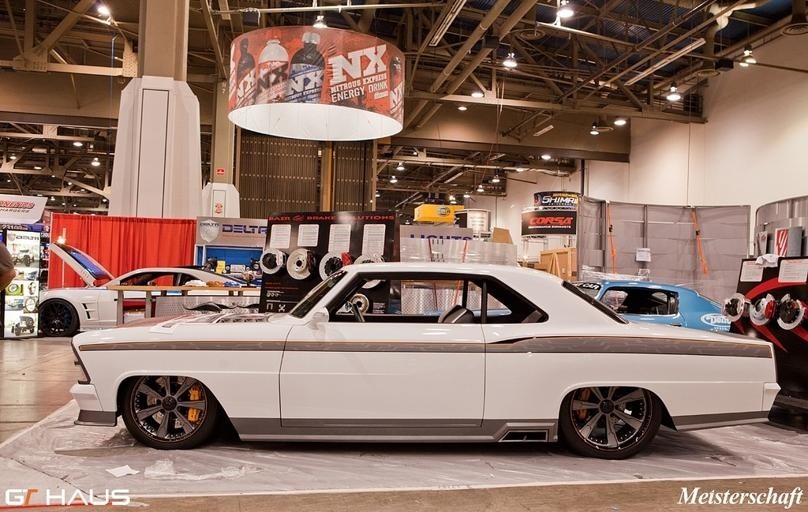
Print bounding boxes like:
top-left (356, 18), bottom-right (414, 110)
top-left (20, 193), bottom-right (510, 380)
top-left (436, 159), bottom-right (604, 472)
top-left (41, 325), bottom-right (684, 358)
top-left (501, 0), bottom-right (576, 70)
top-left (225, 1), bottom-right (406, 141)
top-left (588, 81), bottom-right (681, 136)
top-left (738, 45), bottom-right (759, 68)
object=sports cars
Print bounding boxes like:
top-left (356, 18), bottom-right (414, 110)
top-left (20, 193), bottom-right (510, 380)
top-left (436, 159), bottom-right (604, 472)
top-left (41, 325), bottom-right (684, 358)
top-left (420, 275), bottom-right (743, 343)
top-left (68, 259), bottom-right (784, 462)
top-left (37, 241), bottom-right (264, 339)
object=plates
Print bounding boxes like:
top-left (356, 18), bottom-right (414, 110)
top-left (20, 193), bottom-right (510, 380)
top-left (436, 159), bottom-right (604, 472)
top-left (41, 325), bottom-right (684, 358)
top-left (286, 248), bottom-right (316, 281)
top-left (258, 248), bottom-right (285, 275)
top-left (353, 254), bottom-right (383, 290)
top-left (318, 251), bottom-right (352, 288)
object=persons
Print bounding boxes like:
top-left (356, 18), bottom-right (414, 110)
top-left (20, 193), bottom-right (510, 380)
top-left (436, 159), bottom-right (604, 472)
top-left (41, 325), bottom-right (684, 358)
top-left (0, 241), bottom-right (16, 292)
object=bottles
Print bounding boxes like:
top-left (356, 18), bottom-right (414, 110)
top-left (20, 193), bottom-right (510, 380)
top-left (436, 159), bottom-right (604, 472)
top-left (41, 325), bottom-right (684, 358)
top-left (289, 30), bottom-right (325, 104)
top-left (235, 38), bottom-right (256, 108)
top-left (229, 40), bottom-right (238, 111)
top-left (255, 28), bottom-right (289, 103)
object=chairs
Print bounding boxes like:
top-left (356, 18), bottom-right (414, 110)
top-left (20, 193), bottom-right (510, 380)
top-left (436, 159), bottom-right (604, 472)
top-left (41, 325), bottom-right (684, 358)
top-left (437, 303), bottom-right (543, 324)
